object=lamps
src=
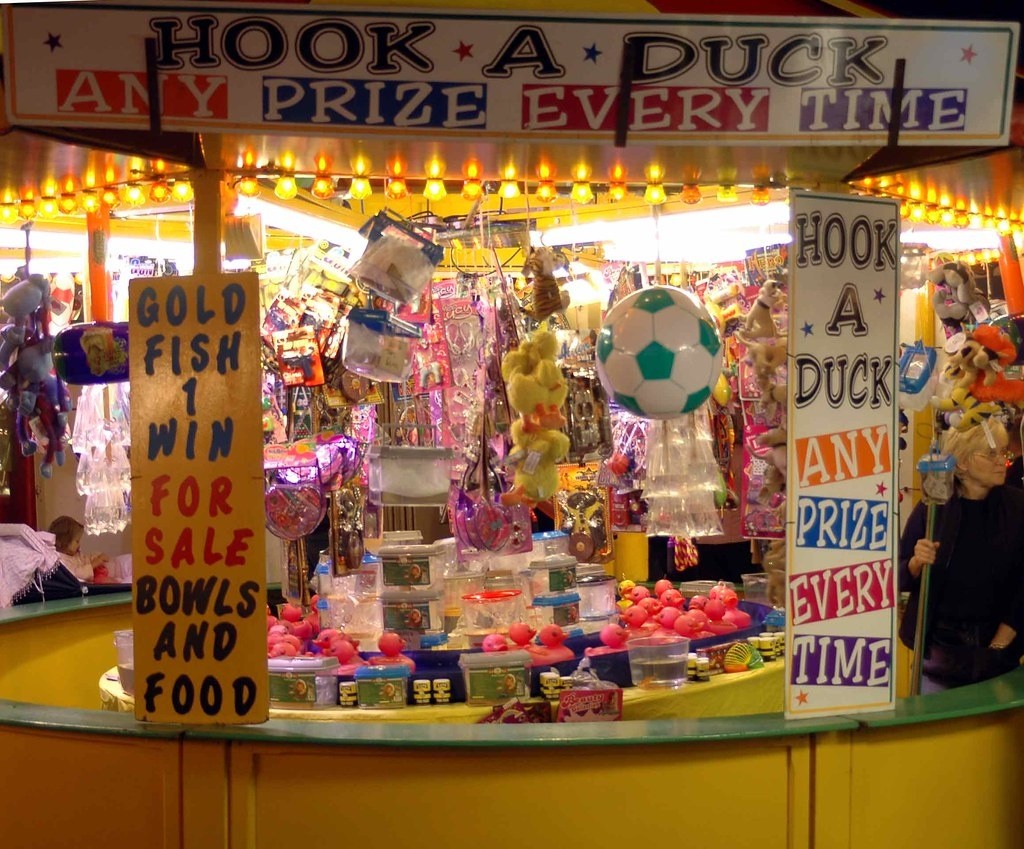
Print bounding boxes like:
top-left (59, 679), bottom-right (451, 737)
top-left (222, 214), bottom-right (266, 262)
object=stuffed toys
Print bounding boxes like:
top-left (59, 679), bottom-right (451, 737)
top-left (735, 278), bottom-right (786, 491)
top-left (487, 330), bottom-right (570, 514)
top-left (916, 266), bottom-right (1024, 424)
top-left (1, 268), bottom-right (71, 477)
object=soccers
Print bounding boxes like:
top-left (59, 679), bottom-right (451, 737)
top-left (594, 284), bottom-right (727, 421)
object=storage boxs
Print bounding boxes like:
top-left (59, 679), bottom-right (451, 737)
top-left (342, 303), bottom-right (421, 384)
top-left (116, 631), bottom-right (134, 695)
top-left (898, 342), bottom-right (937, 412)
top-left (915, 438), bottom-right (955, 505)
top-left (355, 209), bottom-right (447, 305)
top-left (365, 423), bottom-right (454, 508)
top-left (268, 532), bottom-right (769, 709)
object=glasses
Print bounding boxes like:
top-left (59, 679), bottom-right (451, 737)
top-left (972, 448), bottom-right (1014, 461)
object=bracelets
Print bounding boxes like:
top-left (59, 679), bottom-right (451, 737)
top-left (989, 638), bottom-right (1003, 650)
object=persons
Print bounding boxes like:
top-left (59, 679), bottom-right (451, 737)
top-left (894, 418), bottom-right (1024, 687)
top-left (46, 514), bottom-right (108, 584)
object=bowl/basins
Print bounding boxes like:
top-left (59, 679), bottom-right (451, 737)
top-left (269, 545), bottom-right (618, 700)
top-left (627, 636), bottom-right (692, 690)
top-left (742, 572), bottom-right (787, 610)
